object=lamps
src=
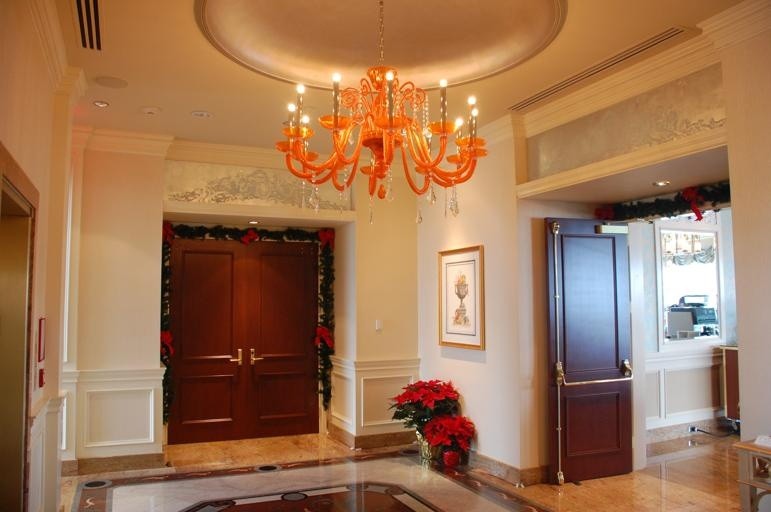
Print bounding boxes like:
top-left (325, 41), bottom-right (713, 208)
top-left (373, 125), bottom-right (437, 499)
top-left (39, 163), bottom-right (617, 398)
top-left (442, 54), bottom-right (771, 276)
top-left (277, 1), bottom-right (490, 224)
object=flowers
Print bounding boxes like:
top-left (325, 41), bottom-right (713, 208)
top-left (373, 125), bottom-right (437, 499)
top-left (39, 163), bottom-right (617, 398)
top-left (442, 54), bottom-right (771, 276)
top-left (386, 379), bottom-right (477, 454)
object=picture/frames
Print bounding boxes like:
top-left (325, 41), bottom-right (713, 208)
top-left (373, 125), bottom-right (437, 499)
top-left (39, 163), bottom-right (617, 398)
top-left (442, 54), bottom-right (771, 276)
top-left (438, 244), bottom-right (487, 351)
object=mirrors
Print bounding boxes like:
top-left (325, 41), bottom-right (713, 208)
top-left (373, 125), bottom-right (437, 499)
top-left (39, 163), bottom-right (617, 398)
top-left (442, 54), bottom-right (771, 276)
top-left (655, 219), bottom-right (727, 352)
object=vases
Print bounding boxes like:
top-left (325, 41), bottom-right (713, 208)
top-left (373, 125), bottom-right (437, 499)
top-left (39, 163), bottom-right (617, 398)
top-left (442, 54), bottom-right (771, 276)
top-left (416, 431), bottom-right (461, 468)
